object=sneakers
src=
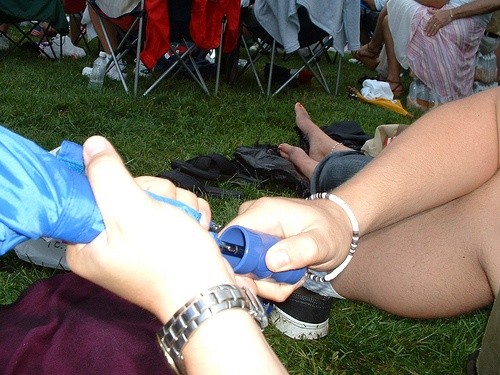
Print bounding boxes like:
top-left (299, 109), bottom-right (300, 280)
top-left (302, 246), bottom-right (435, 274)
top-left (81, 60), bottom-right (128, 81)
top-left (133, 58), bottom-right (154, 78)
top-left (268, 286), bottom-right (332, 340)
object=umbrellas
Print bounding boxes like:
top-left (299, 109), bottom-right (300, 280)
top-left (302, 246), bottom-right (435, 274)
top-left (0, 125), bottom-right (304, 285)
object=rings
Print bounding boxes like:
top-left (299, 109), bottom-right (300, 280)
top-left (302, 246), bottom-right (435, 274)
top-left (434, 24), bottom-right (437, 26)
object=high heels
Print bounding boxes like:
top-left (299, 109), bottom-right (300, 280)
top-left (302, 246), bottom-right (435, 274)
top-left (352, 43), bottom-right (380, 68)
top-left (386, 79), bottom-right (404, 98)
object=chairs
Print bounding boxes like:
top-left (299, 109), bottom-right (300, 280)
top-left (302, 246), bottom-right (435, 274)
top-left (0, 0), bottom-right (382, 103)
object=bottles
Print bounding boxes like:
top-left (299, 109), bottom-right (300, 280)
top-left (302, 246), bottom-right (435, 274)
top-left (409, 78), bottom-right (434, 109)
top-left (88, 51), bottom-right (108, 91)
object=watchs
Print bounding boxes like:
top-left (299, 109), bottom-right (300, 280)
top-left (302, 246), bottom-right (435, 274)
top-left (155, 289), bottom-right (274, 375)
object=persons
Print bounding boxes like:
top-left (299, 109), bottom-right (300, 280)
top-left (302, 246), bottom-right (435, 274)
top-left (0, 0), bottom-right (500, 375)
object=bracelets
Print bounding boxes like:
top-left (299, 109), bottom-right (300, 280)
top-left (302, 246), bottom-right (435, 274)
top-left (305, 193), bottom-right (359, 282)
top-left (449, 9), bottom-right (455, 20)
